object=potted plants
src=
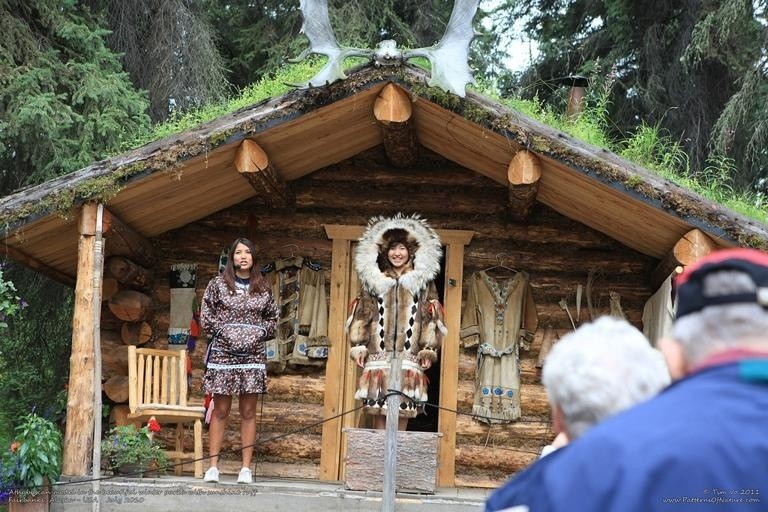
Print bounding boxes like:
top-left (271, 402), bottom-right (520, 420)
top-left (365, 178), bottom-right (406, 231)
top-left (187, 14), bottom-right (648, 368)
top-left (104, 423), bottom-right (168, 479)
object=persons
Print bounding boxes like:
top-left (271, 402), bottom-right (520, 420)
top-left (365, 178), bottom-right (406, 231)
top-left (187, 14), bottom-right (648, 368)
top-left (199, 239), bottom-right (279, 484)
top-left (348, 236), bottom-right (442, 430)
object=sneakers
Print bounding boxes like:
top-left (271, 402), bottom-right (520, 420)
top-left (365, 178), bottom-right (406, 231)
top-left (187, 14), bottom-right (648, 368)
top-left (236, 467), bottom-right (253, 484)
top-left (203, 466), bottom-right (219, 483)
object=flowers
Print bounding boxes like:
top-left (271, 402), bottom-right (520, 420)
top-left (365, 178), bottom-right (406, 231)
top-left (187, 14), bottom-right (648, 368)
top-left (0, 408), bottom-right (64, 504)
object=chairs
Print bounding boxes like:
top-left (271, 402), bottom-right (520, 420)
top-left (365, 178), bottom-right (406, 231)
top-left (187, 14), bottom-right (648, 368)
top-left (127, 344), bottom-right (209, 479)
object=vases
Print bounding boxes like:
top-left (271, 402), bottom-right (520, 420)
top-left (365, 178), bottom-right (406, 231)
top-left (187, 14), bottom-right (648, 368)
top-left (8, 473), bottom-right (50, 512)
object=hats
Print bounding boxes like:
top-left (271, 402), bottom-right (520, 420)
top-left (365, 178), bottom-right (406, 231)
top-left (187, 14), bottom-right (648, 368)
top-left (676, 247), bottom-right (767, 319)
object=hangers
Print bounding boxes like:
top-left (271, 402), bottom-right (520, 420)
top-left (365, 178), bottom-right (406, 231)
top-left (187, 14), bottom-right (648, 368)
top-left (277, 243), bottom-right (297, 262)
top-left (482, 253), bottom-right (518, 280)
